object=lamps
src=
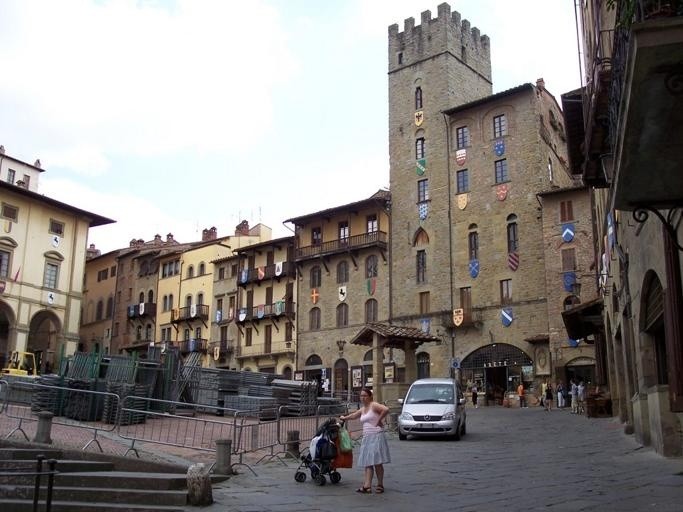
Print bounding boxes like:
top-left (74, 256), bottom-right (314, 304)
top-left (567, 274), bottom-right (606, 297)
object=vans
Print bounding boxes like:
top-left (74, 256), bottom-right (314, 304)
top-left (396, 377), bottom-right (466, 441)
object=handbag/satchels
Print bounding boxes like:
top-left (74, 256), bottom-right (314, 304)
top-left (333, 432), bottom-right (353, 468)
top-left (320, 442), bottom-right (337, 459)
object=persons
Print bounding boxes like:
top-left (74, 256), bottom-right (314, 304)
top-left (340, 388), bottom-right (392, 493)
top-left (471, 383), bottom-right (478, 408)
top-left (539, 377), bottom-right (587, 415)
top-left (517, 382), bottom-right (529, 408)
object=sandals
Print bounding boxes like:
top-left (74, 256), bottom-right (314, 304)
top-left (355, 486), bottom-right (371, 494)
top-left (376, 485), bottom-right (384, 493)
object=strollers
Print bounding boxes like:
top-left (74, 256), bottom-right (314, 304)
top-left (294, 415), bottom-right (345, 486)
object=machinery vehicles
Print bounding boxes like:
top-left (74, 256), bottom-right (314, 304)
top-left (0, 351), bottom-right (55, 376)
